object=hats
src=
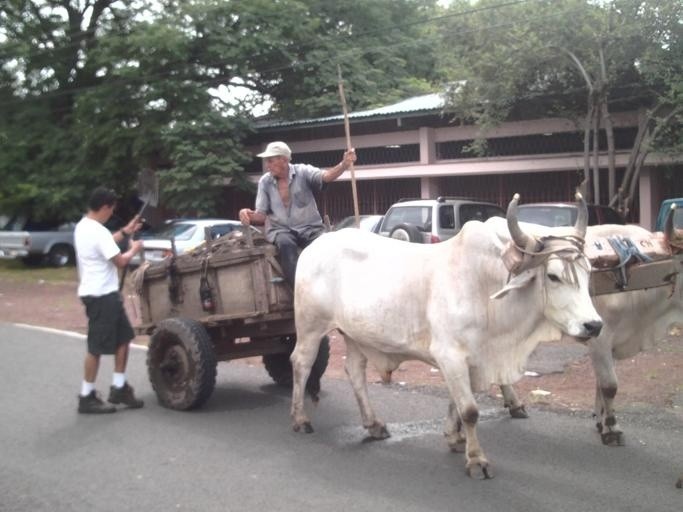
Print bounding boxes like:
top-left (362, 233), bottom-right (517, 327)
top-left (255, 141), bottom-right (291, 161)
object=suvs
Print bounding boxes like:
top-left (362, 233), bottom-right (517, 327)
top-left (377, 195), bottom-right (506, 243)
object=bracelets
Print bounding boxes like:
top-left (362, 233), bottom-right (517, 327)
top-left (121, 229), bottom-right (130, 239)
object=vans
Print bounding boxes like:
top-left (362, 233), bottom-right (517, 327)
top-left (517, 200), bottom-right (627, 227)
top-left (656, 197), bottom-right (683, 234)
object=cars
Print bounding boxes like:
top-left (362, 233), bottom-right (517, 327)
top-left (332, 215), bottom-right (384, 234)
top-left (123, 216), bottom-right (259, 268)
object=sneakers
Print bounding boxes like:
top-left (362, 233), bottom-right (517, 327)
top-left (107, 382), bottom-right (143, 408)
top-left (77, 390), bottom-right (115, 413)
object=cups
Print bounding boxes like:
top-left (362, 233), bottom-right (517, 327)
top-left (199, 287), bottom-right (214, 312)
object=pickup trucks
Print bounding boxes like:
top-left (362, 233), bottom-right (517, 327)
top-left (0, 218), bottom-right (77, 268)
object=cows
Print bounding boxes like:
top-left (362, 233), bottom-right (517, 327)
top-left (483, 202), bottom-right (683, 447)
top-left (289, 191), bottom-right (604, 480)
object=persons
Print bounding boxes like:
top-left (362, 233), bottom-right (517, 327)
top-left (239, 140), bottom-right (357, 291)
top-left (72, 188), bottom-right (145, 413)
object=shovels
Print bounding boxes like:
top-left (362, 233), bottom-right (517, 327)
top-left (119, 167), bottom-right (159, 292)
